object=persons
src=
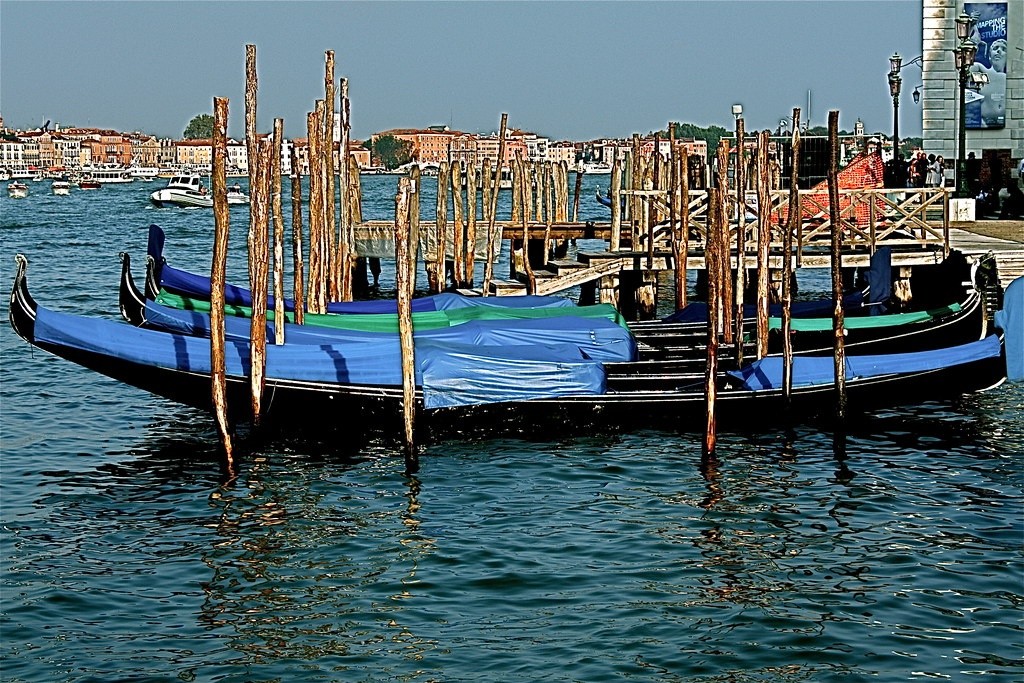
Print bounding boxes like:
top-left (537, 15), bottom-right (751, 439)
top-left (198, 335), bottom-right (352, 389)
top-left (885, 152), bottom-right (977, 205)
top-left (199, 185), bottom-right (207, 195)
top-left (966, 10), bottom-right (1006, 128)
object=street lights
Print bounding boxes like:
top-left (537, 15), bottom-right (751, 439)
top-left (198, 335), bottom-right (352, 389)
top-left (885, 52), bottom-right (906, 202)
top-left (948, 10), bottom-right (979, 222)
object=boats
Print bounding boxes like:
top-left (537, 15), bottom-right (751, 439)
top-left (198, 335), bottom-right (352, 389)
top-left (461, 166), bottom-right (535, 188)
top-left (9, 225), bottom-right (1024, 416)
top-left (584, 164), bottom-right (613, 174)
top-left (150, 172), bottom-right (249, 207)
top-left (51, 181), bottom-right (70, 196)
top-left (78, 179), bottom-right (101, 189)
top-left (0, 161), bottom-right (250, 183)
top-left (7, 182), bottom-right (28, 199)
